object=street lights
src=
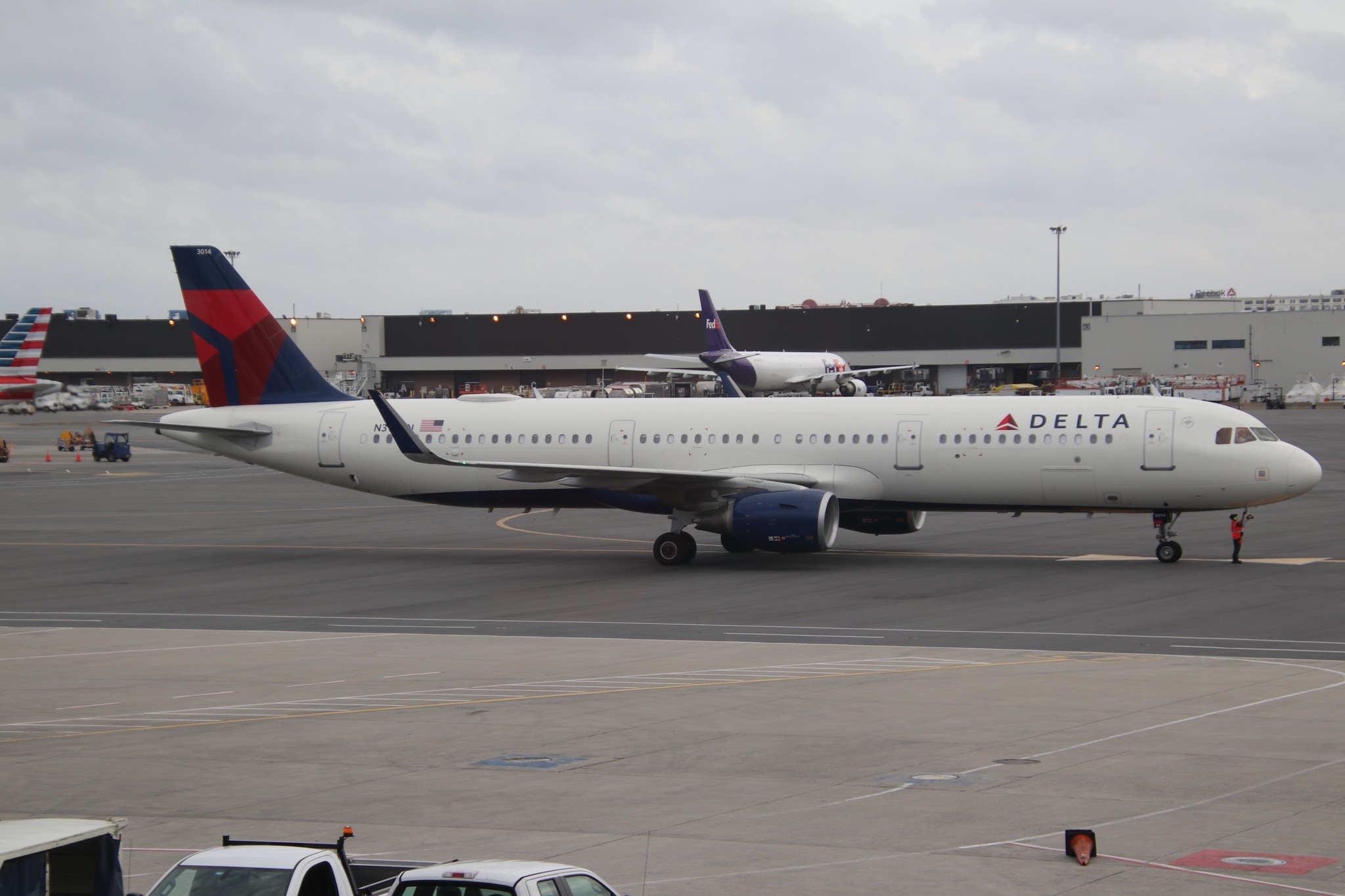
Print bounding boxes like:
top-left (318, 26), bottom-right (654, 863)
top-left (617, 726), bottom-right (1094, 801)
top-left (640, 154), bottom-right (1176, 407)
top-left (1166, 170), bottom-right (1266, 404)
top-left (1049, 226), bottom-right (1068, 379)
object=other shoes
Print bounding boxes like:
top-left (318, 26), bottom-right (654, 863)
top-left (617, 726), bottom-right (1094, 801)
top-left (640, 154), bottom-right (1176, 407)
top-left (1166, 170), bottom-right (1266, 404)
top-left (1232, 560), bottom-right (1242, 564)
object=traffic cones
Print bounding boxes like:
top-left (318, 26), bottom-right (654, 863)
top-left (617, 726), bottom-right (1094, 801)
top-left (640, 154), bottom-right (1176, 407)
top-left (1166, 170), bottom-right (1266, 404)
top-left (74, 450), bottom-right (82, 462)
top-left (45, 449), bottom-right (51, 462)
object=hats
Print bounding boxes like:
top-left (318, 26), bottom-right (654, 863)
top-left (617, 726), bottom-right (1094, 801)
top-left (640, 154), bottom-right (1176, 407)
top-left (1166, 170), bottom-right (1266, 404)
top-left (1230, 514), bottom-right (1238, 520)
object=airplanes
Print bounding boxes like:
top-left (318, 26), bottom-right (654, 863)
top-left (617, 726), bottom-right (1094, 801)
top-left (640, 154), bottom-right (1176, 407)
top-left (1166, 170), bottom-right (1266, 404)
top-left (0, 307), bottom-right (63, 414)
top-left (153, 245), bottom-right (1324, 567)
top-left (613, 288), bottom-right (868, 397)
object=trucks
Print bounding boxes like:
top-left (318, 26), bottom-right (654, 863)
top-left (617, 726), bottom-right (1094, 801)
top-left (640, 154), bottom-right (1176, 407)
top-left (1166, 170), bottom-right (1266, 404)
top-left (3, 383), bottom-right (194, 415)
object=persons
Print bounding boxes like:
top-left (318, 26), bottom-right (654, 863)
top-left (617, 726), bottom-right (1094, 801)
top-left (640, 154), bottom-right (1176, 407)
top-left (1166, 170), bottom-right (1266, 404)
top-left (1230, 511), bottom-right (1250, 563)
